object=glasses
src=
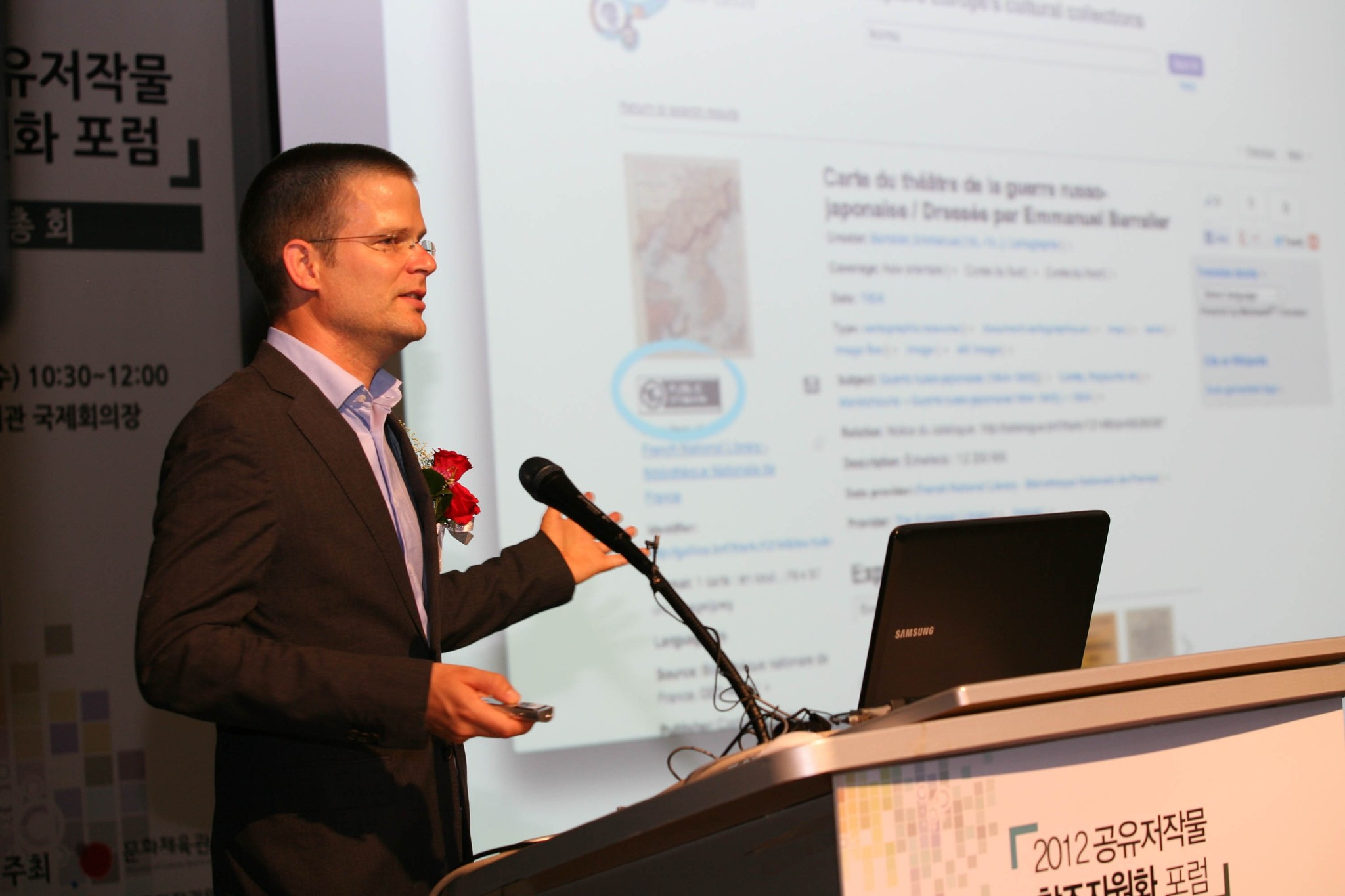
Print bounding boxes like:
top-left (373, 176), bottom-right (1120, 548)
top-left (305, 231), bottom-right (436, 258)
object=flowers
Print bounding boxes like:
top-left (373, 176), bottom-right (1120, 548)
top-left (398, 416), bottom-right (481, 554)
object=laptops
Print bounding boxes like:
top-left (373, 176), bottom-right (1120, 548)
top-left (857, 510), bottom-right (1111, 718)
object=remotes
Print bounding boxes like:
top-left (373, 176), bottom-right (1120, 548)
top-left (480, 696), bottom-right (554, 723)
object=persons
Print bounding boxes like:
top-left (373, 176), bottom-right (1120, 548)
top-left (133, 138), bottom-right (652, 896)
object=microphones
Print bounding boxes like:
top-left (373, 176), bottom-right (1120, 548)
top-left (519, 455), bottom-right (771, 746)
